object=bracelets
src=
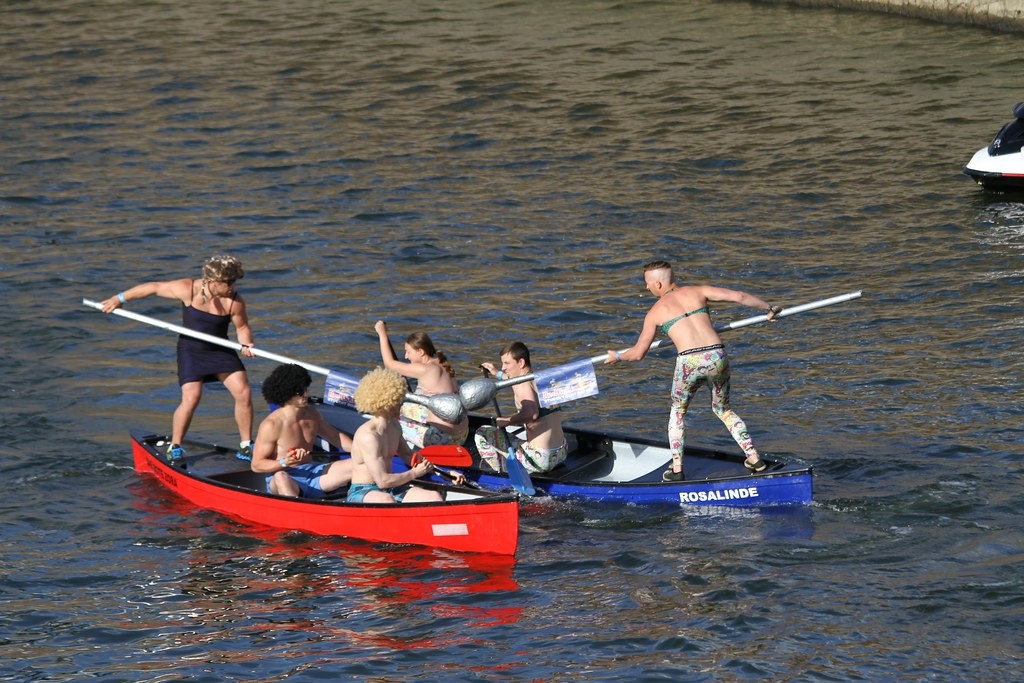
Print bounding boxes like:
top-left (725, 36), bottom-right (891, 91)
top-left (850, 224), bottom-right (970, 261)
top-left (117, 291), bottom-right (128, 304)
top-left (496, 371), bottom-right (503, 381)
top-left (279, 459), bottom-right (286, 467)
top-left (615, 353), bottom-right (619, 360)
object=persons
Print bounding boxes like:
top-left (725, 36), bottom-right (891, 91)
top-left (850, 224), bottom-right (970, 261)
top-left (347, 368), bottom-right (466, 503)
top-left (101, 255), bottom-right (256, 461)
top-left (604, 260), bottom-right (784, 482)
top-left (474, 342), bottom-right (569, 475)
top-left (251, 364), bottom-right (354, 501)
top-left (375, 319), bottom-right (468, 449)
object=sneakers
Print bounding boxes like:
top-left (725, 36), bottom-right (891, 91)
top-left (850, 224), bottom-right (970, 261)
top-left (662, 470), bottom-right (685, 481)
top-left (166, 444), bottom-right (187, 462)
top-left (744, 459), bottom-right (766, 471)
top-left (237, 441), bottom-right (255, 460)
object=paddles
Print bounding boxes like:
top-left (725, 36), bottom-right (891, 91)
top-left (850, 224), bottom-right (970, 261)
top-left (479, 364), bottom-right (535, 495)
top-left (288, 444), bottom-right (473, 467)
top-left (384, 322), bottom-right (413, 393)
top-left (410, 451), bottom-right (477, 489)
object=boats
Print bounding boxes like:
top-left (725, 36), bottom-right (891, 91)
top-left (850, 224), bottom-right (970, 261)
top-left (130, 396), bottom-right (814, 557)
top-left (964, 101), bottom-right (1024, 191)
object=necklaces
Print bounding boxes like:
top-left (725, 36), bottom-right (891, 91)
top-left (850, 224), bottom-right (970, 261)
top-left (663, 287), bottom-right (676, 295)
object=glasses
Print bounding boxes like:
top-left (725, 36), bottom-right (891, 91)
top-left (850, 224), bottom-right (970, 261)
top-left (219, 278), bottom-right (235, 286)
